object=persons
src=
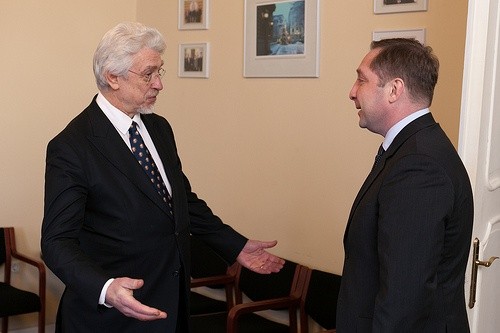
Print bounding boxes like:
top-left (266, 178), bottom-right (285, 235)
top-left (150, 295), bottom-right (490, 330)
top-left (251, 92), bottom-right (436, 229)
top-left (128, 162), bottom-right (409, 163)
top-left (40, 21), bottom-right (285, 333)
top-left (335, 37), bottom-right (470, 333)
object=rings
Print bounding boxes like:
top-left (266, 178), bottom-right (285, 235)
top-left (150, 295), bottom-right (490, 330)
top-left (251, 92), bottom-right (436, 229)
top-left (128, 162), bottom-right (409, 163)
top-left (260, 264), bottom-right (265, 269)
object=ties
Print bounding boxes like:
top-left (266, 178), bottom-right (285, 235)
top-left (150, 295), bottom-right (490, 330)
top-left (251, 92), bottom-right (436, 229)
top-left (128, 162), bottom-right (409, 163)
top-left (374, 144), bottom-right (385, 168)
top-left (128, 122), bottom-right (174, 217)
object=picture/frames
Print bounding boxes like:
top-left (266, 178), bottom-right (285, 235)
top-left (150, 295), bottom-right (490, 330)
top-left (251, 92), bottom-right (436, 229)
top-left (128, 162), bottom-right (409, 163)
top-left (243, 0), bottom-right (320, 78)
top-left (178, 42), bottom-right (209, 78)
top-left (373, 0), bottom-right (427, 14)
top-left (178, 0), bottom-right (209, 30)
top-left (372, 29), bottom-right (426, 47)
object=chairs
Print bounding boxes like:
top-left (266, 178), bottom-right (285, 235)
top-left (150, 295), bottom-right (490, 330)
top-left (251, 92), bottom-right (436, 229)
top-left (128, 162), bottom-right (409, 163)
top-left (188, 236), bottom-right (343, 333)
top-left (0, 227), bottom-right (46, 333)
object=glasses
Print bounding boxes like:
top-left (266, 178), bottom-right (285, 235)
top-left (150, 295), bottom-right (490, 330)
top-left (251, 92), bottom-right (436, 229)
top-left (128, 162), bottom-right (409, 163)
top-left (128, 67), bottom-right (164, 83)
